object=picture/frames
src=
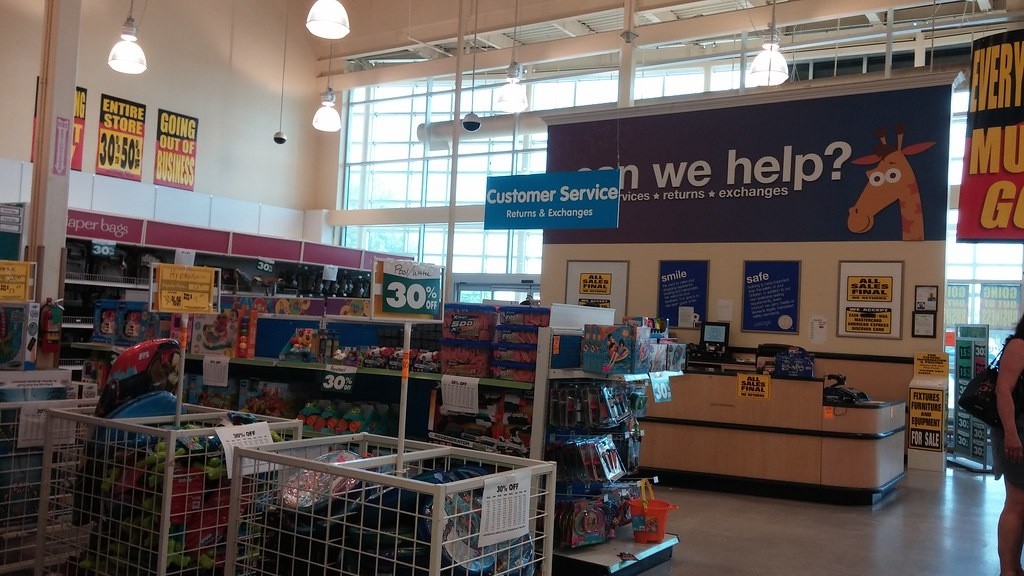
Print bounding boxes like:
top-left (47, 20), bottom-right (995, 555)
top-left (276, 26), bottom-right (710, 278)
top-left (741, 259), bottom-right (802, 335)
top-left (835, 260), bottom-right (906, 340)
top-left (914, 285), bottom-right (938, 312)
top-left (656, 259), bottom-right (711, 329)
top-left (565, 259), bottom-right (631, 325)
top-left (911, 311), bottom-right (936, 338)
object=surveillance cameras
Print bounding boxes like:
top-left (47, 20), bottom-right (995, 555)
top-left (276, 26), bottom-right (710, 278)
top-left (274, 132), bottom-right (287, 144)
top-left (463, 114), bottom-right (482, 132)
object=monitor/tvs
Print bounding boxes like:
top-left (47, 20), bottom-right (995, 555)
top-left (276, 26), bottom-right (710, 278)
top-left (755, 343), bottom-right (806, 374)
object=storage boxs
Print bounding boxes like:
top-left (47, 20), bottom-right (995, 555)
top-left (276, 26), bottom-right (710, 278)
top-left (238, 379), bottom-right (298, 419)
top-left (597, 325), bottom-right (633, 373)
top-left (441, 303), bottom-right (499, 342)
top-left (317, 328), bottom-right (333, 362)
top-left (188, 374), bottom-right (240, 409)
top-left (169, 312), bottom-right (194, 352)
top-left (333, 329), bottom-right (341, 354)
top-left (497, 306), bottom-right (551, 326)
top-left (412, 349), bottom-right (441, 373)
top-left (440, 338), bottom-right (494, 378)
top-left (91, 299), bottom-right (119, 343)
top-left (310, 383), bottom-right (325, 399)
top-left (299, 382), bottom-right (312, 402)
top-left (496, 325), bottom-right (538, 344)
top-left (589, 324), bottom-right (598, 372)
top-left (388, 347), bottom-right (419, 370)
top-left (492, 341), bottom-right (538, 363)
top-left (631, 326), bottom-right (652, 374)
top-left (622, 316), bottom-right (670, 338)
top-left (649, 338), bottom-right (667, 371)
top-left (362, 346), bottom-right (396, 368)
top-left (582, 324), bottom-right (590, 372)
top-left (115, 300), bottom-right (171, 347)
top-left (659, 337), bottom-right (687, 371)
top-left (426, 381), bottom-right (534, 458)
top-left (494, 360), bottom-right (536, 383)
top-left (190, 308), bottom-right (258, 360)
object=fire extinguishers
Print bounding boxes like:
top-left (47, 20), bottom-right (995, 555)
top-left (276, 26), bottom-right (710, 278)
top-left (38, 297), bottom-right (65, 354)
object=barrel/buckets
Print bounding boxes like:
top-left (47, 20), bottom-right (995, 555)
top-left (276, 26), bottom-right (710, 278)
top-left (628, 478), bottom-right (679, 544)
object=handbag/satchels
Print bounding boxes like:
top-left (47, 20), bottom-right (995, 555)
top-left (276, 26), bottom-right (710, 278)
top-left (957, 337), bottom-right (1024, 427)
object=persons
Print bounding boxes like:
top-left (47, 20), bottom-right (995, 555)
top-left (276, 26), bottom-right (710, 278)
top-left (638, 340), bottom-right (648, 365)
top-left (605, 333), bottom-right (627, 368)
top-left (990, 313), bottom-right (1024, 575)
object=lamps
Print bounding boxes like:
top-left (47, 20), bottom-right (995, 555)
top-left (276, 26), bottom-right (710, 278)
top-left (495, 0), bottom-right (528, 113)
top-left (107, 0), bottom-right (147, 74)
top-left (311, 39), bottom-right (342, 133)
top-left (305, 0), bottom-right (350, 40)
top-left (746, 0), bottom-right (789, 86)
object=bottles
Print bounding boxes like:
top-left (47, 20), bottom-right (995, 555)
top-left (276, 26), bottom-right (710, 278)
top-left (672, 345), bottom-right (682, 365)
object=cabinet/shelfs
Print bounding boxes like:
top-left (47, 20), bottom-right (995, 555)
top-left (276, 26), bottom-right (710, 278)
top-left (0, 234), bottom-right (686, 576)
